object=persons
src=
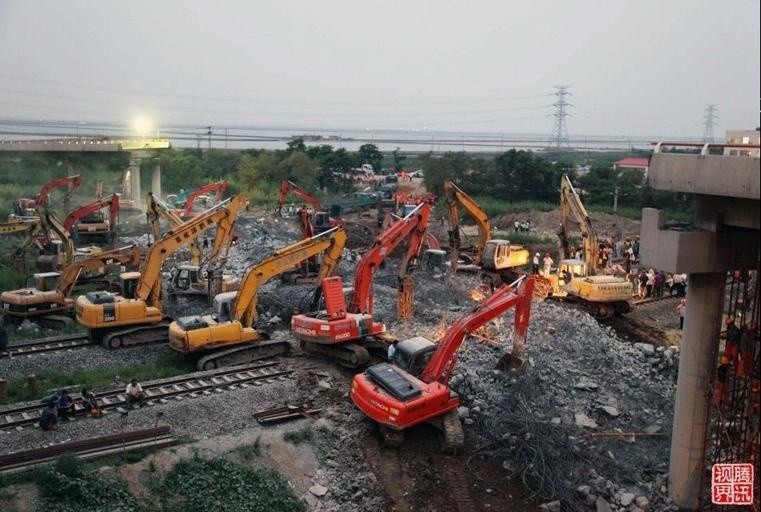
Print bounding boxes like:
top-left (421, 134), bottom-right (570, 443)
top-left (56, 389), bottom-right (77, 421)
top-left (125, 378), bottom-right (147, 410)
top-left (245, 199), bottom-right (250, 210)
top-left (298, 166), bottom-right (439, 228)
top-left (511, 218), bottom-right (760, 422)
top-left (166, 278), bottom-right (179, 304)
top-left (387, 339), bottom-right (400, 364)
top-left (80, 388), bottom-right (103, 417)
top-left (203, 235), bottom-right (209, 248)
top-left (201, 193), bottom-right (215, 211)
top-left (231, 235), bottom-right (240, 246)
top-left (39, 401), bottom-right (59, 431)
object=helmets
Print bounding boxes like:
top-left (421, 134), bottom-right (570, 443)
top-left (726, 319), bottom-right (732, 323)
top-left (720, 356), bottom-right (729, 364)
